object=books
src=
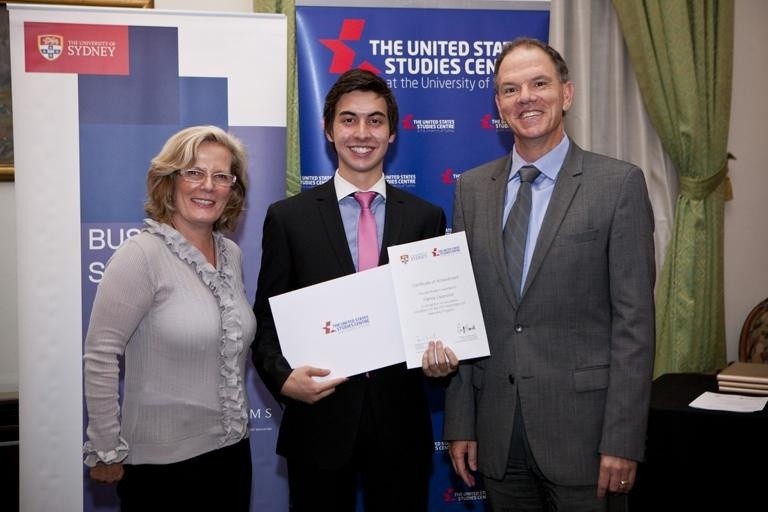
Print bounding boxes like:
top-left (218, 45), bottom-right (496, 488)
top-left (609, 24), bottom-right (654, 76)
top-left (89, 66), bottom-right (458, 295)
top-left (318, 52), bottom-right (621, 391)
top-left (716, 361), bottom-right (768, 396)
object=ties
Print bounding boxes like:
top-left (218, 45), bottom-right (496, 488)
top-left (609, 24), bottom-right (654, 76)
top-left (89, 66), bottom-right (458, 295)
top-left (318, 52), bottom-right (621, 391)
top-left (354, 192), bottom-right (379, 272)
top-left (502, 168), bottom-right (542, 303)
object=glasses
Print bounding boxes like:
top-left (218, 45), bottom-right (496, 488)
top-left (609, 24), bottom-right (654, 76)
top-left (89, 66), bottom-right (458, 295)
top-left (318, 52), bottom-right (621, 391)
top-left (176, 170), bottom-right (236, 186)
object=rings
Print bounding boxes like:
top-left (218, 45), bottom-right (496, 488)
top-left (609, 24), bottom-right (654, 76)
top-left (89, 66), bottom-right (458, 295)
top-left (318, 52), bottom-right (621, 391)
top-left (620, 480), bottom-right (629, 485)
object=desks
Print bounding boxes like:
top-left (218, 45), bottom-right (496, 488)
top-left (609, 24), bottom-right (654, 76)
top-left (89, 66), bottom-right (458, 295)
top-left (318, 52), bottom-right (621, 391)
top-left (626, 371), bottom-right (767, 512)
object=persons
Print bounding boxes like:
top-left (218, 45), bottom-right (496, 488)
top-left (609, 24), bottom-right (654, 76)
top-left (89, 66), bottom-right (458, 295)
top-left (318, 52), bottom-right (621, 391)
top-left (83, 125), bottom-right (257, 511)
top-left (251, 68), bottom-right (459, 512)
top-left (450, 36), bottom-right (657, 509)
